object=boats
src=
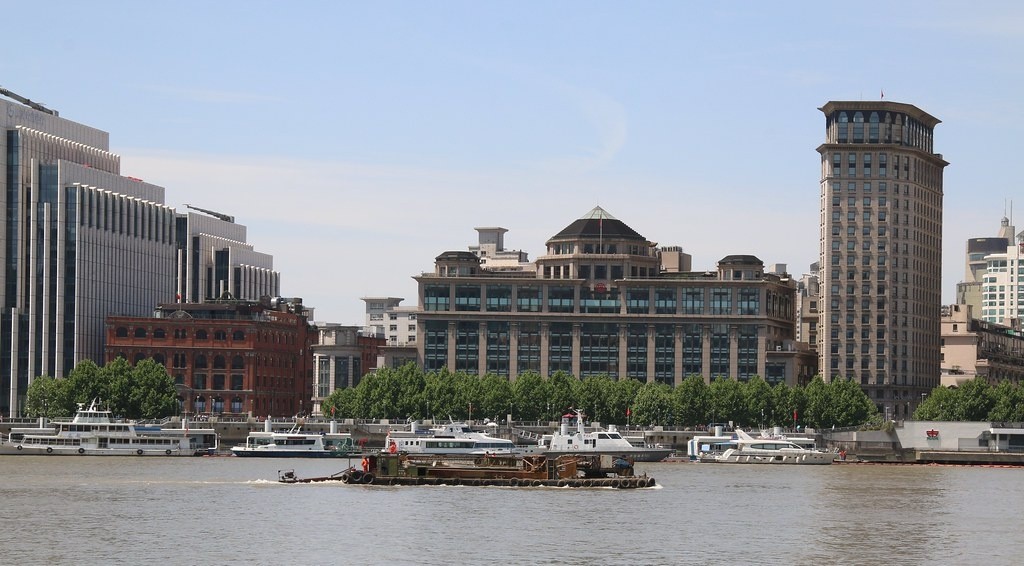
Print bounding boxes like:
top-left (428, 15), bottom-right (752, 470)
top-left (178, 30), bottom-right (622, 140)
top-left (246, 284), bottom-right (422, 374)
top-left (380, 419), bottom-right (546, 457)
top-left (0, 397), bottom-right (220, 458)
top-left (229, 418), bottom-right (363, 460)
top-left (276, 452), bottom-right (659, 490)
top-left (685, 423), bottom-right (839, 465)
top-left (537, 408), bottom-right (679, 464)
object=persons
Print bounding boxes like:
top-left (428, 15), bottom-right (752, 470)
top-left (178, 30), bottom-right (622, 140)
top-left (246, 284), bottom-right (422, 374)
top-left (361, 457), bottom-right (370, 474)
